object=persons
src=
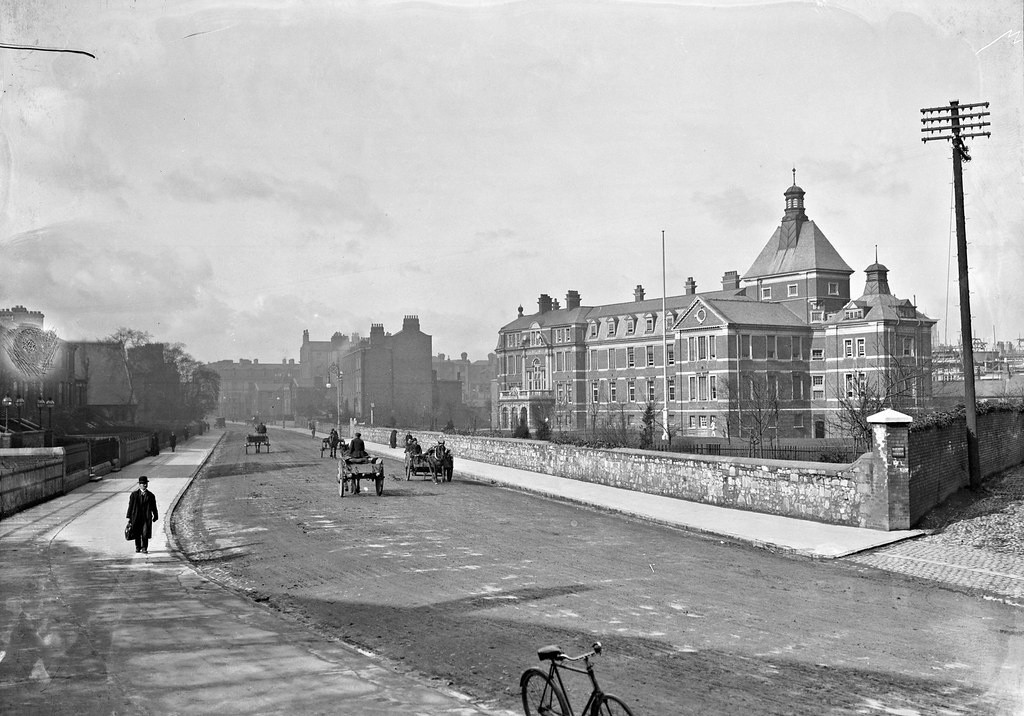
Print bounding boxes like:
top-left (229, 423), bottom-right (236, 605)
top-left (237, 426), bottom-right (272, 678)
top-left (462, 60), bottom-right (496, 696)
top-left (403, 431), bottom-right (422, 465)
top-left (126, 476), bottom-right (158, 553)
top-left (330, 429), bottom-right (338, 459)
top-left (184, 426), bottom-right (189, 441)
top-left (151, 435), bottom-right (159, 455)
top-left (349, 433), bottom-right (364, 459)
top-left (255, 422), bottom-right (266, 434)
top-left (312, 425), bottom-right (315, 438)
top-left (170, 432), bottom-right (176, 452)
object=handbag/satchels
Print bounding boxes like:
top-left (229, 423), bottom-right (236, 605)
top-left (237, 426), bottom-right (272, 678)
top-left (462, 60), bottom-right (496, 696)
top-left (124, 521), bottom-right (136, 541)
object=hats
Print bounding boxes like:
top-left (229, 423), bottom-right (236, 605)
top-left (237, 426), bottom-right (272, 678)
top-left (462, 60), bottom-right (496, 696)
top-left (407, 431), bottom-right (410, 435)
top-left (407, 439), bottom-right (412, 445)
top-left (413, 438), bottom-right (417, 442)
top-left (137, 476), bottom-right (149, 484)
top-left (171, 431), bottom-right (175, 434)
top-left (355, 433), bottom-right (361, 436)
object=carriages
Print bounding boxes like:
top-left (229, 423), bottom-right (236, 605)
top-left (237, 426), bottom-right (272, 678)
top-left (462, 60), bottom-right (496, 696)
top-left (244, 426), bottom-right (270, 455)
top-left (335, 442), bottom-right (385, 497)
top-left (319, 431), bottom-right (347, 459)
top-left (403, 439), bottom-right (453, 486)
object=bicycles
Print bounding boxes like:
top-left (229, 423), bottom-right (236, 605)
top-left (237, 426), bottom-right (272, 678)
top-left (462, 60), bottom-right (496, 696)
top-left (519, 640), bottom-right (634, 716)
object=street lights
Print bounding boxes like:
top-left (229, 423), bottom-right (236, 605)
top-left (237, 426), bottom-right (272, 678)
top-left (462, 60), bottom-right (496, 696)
top-left (327, 361), bottom-right (343, 439)
top-left (2, 393), bottom-right (55, 432)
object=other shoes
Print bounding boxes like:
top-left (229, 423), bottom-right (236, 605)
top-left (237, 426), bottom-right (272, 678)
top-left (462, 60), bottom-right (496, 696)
top-left (142, 547), bottom-right (148, 554)
top-left (135, 548), bottom-right (141, 553)
